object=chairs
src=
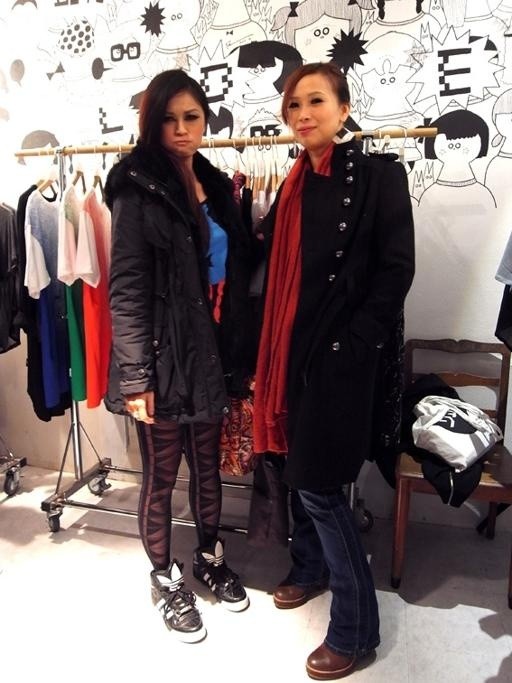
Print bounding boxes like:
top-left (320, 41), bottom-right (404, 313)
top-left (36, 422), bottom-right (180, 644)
top-left (389, 338), bottom-right (512, 590)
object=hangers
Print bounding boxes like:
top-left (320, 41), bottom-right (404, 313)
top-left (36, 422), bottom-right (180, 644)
top-left (34, 125), bottom-right (425, 197)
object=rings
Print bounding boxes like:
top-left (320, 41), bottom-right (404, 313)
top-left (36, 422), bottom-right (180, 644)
top-left (130, 410), bottom-right (140, 419)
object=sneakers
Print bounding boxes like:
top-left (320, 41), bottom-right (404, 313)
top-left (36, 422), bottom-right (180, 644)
top-left (193, 538), bottom-right (249, 612)
top-left (150, 560), bottom-right (206, 642)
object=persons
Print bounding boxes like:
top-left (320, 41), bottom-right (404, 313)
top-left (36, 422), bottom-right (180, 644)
top-left (241, 58), bottom-right (421, 681)
top-left (101, 68), bottom-right (264, 648)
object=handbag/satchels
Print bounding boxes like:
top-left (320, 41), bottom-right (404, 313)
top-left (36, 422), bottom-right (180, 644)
top-left (412, 394), bottom-right (503, 472)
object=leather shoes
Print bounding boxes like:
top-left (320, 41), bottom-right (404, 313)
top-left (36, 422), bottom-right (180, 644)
top-left (274, 576), bottom-right (315, 610)
top-left (306, 641), bottom-right (356, 680)
